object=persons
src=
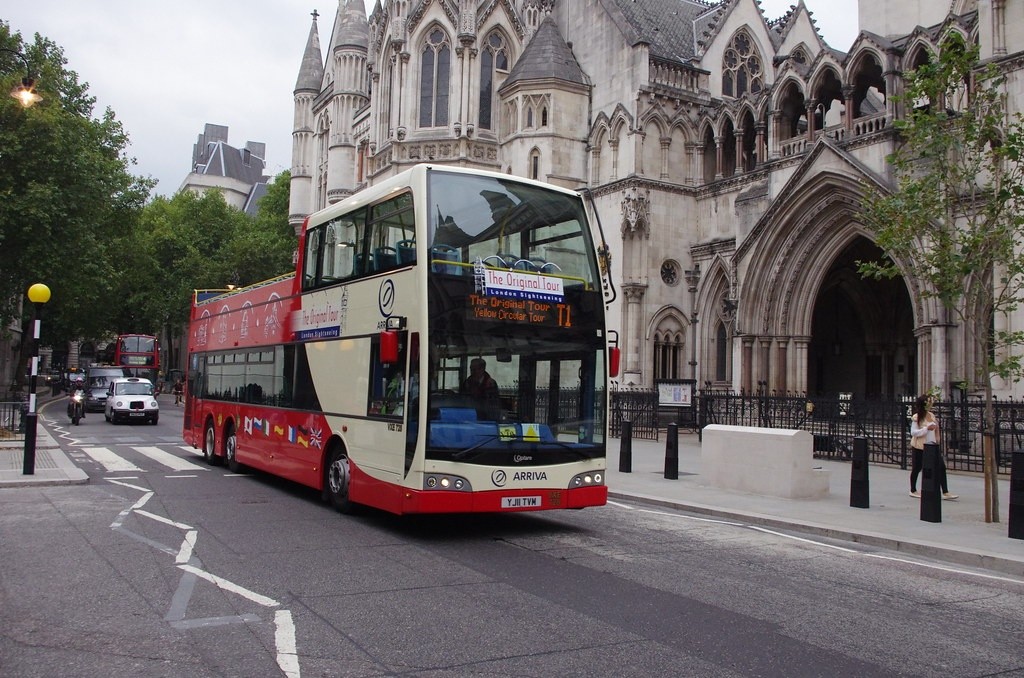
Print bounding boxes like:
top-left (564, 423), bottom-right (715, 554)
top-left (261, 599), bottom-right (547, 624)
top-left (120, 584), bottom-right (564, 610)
top-left (464, 358), bottom-right (500, 408)
top-left (68, 369), bottom-right (89, 418)
top-left (175, 380), bottom-right (183, 404)
top-left (909, 395), bottom-right (960, 501)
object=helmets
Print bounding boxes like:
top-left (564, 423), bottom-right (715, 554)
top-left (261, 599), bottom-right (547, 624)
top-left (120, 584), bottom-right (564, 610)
top-left (76, 377), bottom-right (84, 386)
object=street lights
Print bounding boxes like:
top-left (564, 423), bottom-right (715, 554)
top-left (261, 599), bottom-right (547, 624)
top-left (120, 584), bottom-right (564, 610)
top-left (21, 282), bottom-right (51, 475)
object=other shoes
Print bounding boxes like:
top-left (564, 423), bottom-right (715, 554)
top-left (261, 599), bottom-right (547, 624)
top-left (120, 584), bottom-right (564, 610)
top-left (943, 494), bottom-right (960, 500)
top-left (82, 414), bottom-right (86, 418)
top-left (180, 400), bottom-right (182, 402)
top-left (175, 401), bottom-right (177, 404)
top-left (910, 491), bottom-right (921, 497)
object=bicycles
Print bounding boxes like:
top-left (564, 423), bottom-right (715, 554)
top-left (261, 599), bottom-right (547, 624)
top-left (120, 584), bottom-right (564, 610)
top-left (173, 390), bottom-right (184, 408)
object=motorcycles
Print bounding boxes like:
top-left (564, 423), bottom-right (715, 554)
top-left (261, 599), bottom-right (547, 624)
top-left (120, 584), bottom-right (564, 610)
top-left (65, 393), bottom-right (93, 423)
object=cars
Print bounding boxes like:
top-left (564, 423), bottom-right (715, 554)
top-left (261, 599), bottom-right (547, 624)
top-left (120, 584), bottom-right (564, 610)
top-left (45, 367), bottom-right (61, 383)
top-left (105, 377), bottom-right (160, 425)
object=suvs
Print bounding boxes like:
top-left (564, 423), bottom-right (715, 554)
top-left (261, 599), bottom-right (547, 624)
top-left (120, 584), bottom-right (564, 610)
top-left (63, 369), bottom-right (86, 382)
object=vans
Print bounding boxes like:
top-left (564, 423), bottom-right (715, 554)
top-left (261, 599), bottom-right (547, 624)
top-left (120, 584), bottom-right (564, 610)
top-left (84, 365), bottom-right (124, 413)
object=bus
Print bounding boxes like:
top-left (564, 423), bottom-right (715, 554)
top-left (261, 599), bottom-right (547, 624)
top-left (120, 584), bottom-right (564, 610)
top-left (183, 160), bottom-right (624, 528)
top-left (112, 333), bottom-right (162, 398)
